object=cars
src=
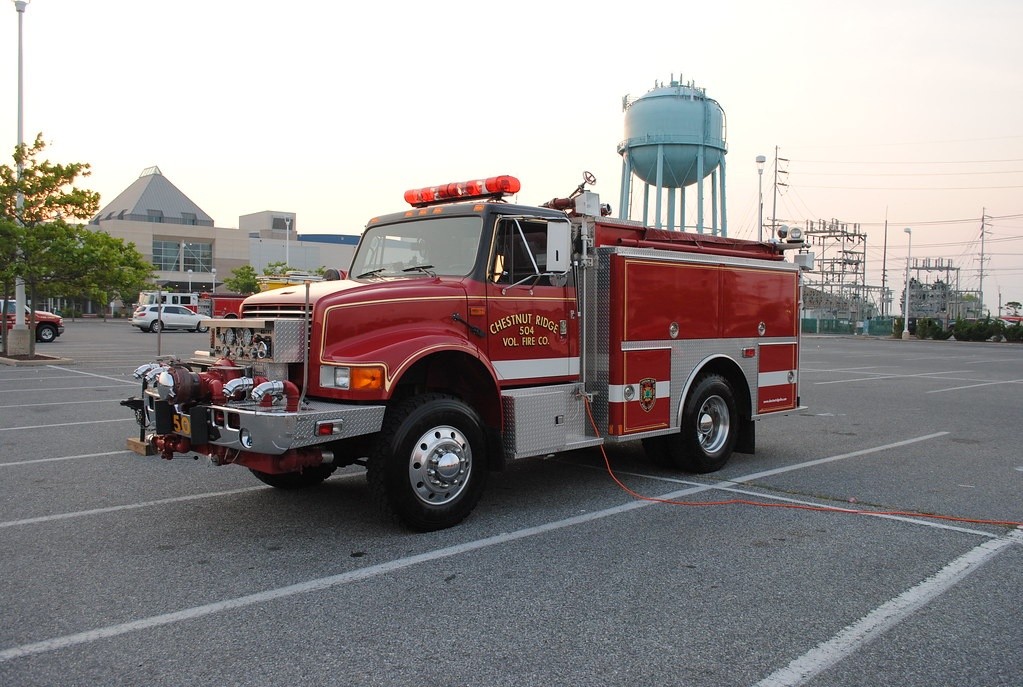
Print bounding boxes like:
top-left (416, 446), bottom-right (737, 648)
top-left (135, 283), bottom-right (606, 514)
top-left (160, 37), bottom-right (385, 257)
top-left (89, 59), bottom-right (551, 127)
top-left (0, 299), bottom-right (64, 343)
top-left (131, 303), bottom-right (212, 332)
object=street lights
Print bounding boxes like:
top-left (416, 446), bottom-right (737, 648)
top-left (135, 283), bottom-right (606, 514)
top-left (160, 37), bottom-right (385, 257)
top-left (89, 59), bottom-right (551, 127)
top-left (4, 0), bottom-right (33, 358)
top-left (900, 229), bottom-right (912, 340)
top-left (754, 155), bottom-right (767, 243)
top-left (283, 215), bottom-right (291, 268)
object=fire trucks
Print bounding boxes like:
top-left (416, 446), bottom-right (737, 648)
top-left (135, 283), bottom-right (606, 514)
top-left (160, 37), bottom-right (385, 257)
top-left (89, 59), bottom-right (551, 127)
top-left (120, 169), bottom-right (815, 532)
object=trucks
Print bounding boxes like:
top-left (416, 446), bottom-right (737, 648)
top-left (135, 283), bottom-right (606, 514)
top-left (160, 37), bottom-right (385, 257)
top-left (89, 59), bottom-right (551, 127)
top-left (136, 289), bottom-right (254, 320)
